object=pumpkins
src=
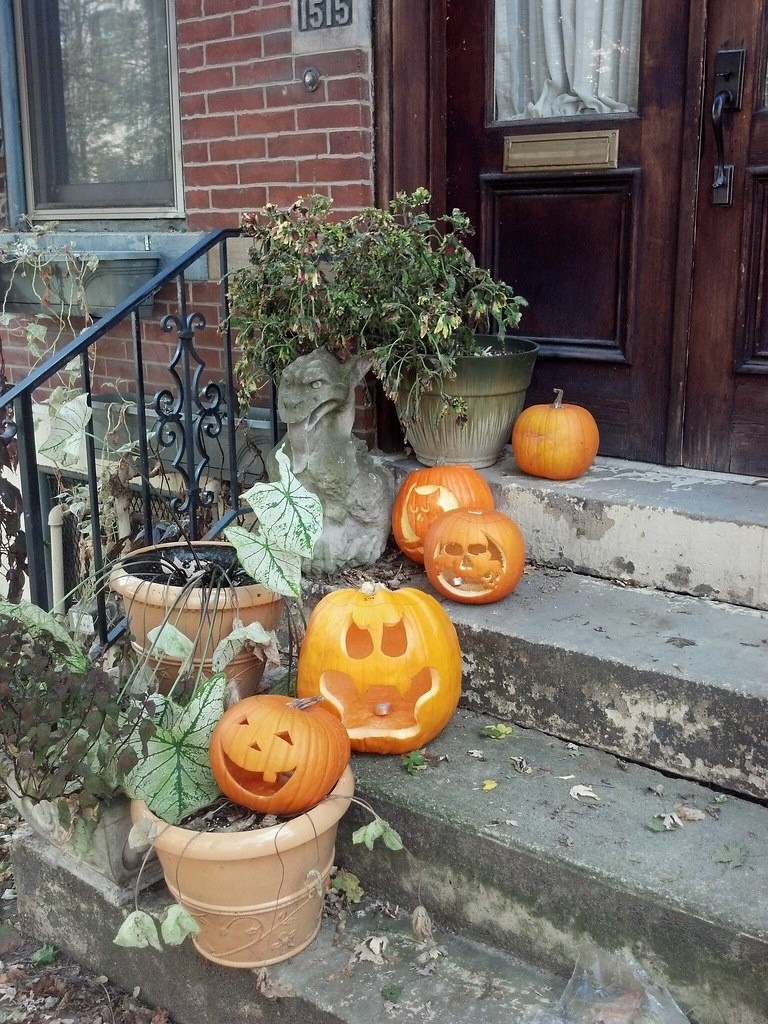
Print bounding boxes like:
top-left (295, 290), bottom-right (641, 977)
top-left (511, 388), bottom-right (599, 479)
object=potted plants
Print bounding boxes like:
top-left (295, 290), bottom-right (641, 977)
top-left (129, 758), bottom-right (358, 970)
top-left (239, 187), bottom-right (541, 475)
top-left (0, 540), bottom-right (283, 847)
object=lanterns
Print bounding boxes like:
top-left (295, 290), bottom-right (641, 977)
top-left (296, 581), bottom-right (465, 755)
top-left (207, 691), bottom-right (352, 813)
top-left (390, 465), bottom-right (494, 565)
top-left (422, 508), bottom-right (527, 607)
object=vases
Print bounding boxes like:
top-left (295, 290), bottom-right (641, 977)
top-left (0, 251), bottom-right (160, 322)
top-left (80, 392), bottom-right (293, 488)
